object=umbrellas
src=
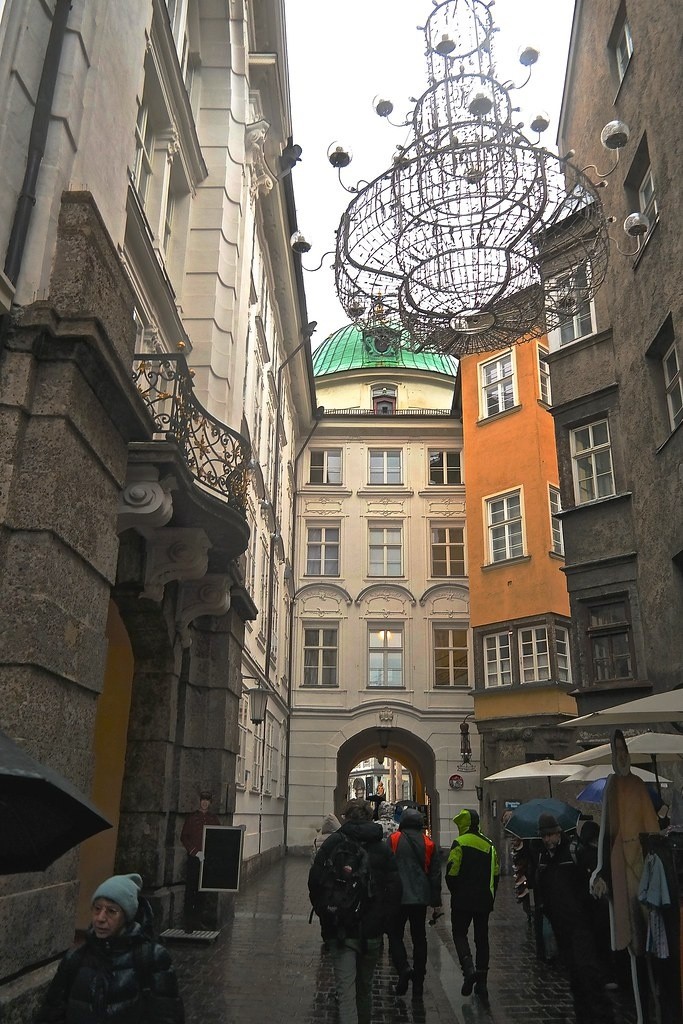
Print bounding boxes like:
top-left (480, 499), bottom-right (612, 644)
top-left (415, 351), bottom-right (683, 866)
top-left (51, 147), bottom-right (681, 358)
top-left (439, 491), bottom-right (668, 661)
top-left (484, 757), bottom-right (587, 798)
top-left (548, 732), bottom-right (683, 796)
top-left (0, 732), bottom-right (115, 875)
top-left (576, 778), bottom-right (665, 809)
top-left (505, 799), bottom-right (582, 840)
top-left (395, 799), bottom-right (421, 807)
top-left (557, 689), bottom-right (683, 726)
top-left (560, 765), bottom-right (675, 783)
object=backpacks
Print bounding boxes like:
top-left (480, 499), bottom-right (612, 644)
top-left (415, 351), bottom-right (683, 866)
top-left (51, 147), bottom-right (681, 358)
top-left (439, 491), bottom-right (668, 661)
top-left (316, 833), bottom-right (372, 928)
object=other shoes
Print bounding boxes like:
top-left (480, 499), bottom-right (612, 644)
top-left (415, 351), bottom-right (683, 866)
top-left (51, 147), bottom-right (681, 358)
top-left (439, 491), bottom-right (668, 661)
top-left (602, 981), bottom-right (617, 989)
top-left (396, 967), bottom-right (411, 995)
top-left (461, 967), bottom-right (474, 996)
top-left (412, 983), bottom-right (424, 994)
top-left (475, 980), bottom-right (487, 994)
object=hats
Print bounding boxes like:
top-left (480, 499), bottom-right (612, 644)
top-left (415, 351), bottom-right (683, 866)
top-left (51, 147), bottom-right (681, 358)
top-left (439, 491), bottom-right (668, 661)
top-left (90, 873), bottom-right (143, 922)
top-left (539, 814), bottom-right (561, 835)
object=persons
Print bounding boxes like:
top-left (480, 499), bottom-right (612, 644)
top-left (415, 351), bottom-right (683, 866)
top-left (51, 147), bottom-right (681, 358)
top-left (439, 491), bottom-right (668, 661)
top-left (394, 806), bottom-right (403, 823)
top-left (181, 792), bottom-right (246, 934)
top-left (384, 808), bottom-right (443, 997)
top-left (539, 813), bottom-right (568, 864)
top-left (365, 783), bottom-right (386, 821)
top-left (311, 813), bottom-right (342, 866)
top-left (445, 809), bottom-right (500, 999)
top-left (34, 871), bottom-right (185, 1024)
top-left (593, 731), bottom-right (662, 1024)
top-left (374, 800), bottom-right (401, 842)
top-left (308, 799), bottom-right (399, 1024)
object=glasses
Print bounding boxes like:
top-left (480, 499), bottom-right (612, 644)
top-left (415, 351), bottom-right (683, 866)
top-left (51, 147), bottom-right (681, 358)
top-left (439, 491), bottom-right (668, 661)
top-left (93, 904), bottom-right (122, 917)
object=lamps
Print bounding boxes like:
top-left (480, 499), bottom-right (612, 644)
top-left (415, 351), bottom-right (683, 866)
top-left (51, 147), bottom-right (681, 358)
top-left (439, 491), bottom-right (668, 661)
top-left (288, 1), bottom-right (649, 362)
top-left (240, 674), bottom-right (275, 723)
top-left (457, 711), bottom-right (477, 774)
